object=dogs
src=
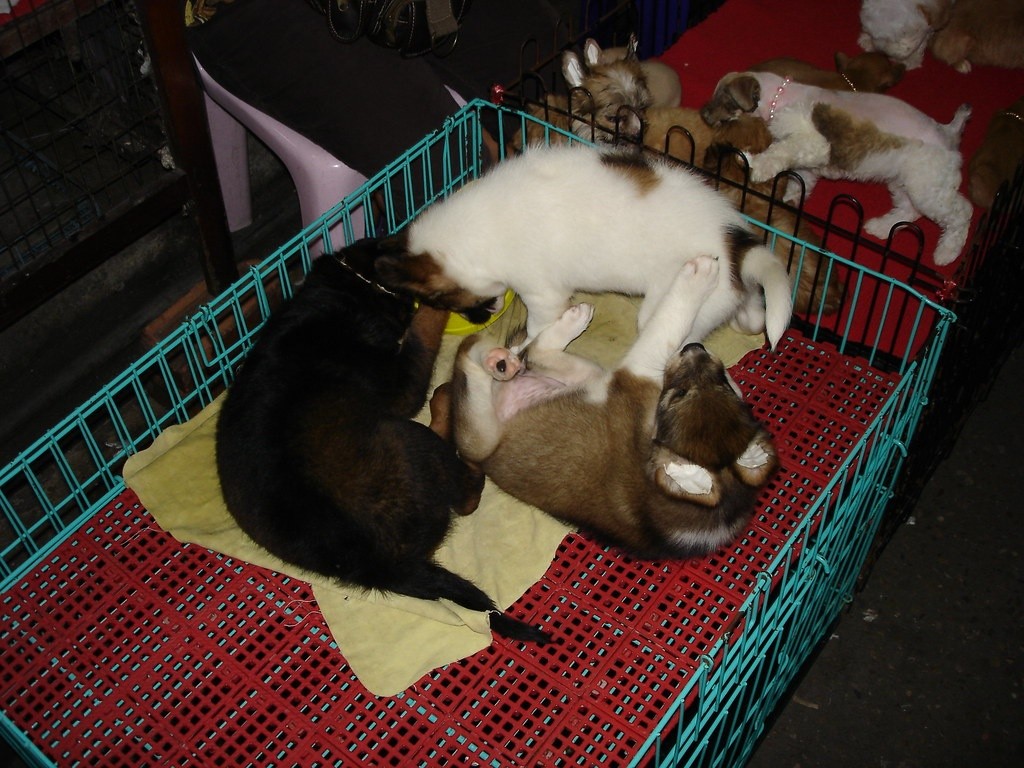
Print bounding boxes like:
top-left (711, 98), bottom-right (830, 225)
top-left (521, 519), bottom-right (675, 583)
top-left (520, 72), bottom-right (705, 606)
top-left (215, 233), bottom-right (552, 646)
top-left (702, 72), bottom-right (973, 267)
top-left (858, 0), bottom-right (1024, 74)
top-left (444, 32), bottom-right (907, 314)
top-left (369, 141), bottom-right (794, 366)
top-left (452, 257), bottom-right (775, 558)
